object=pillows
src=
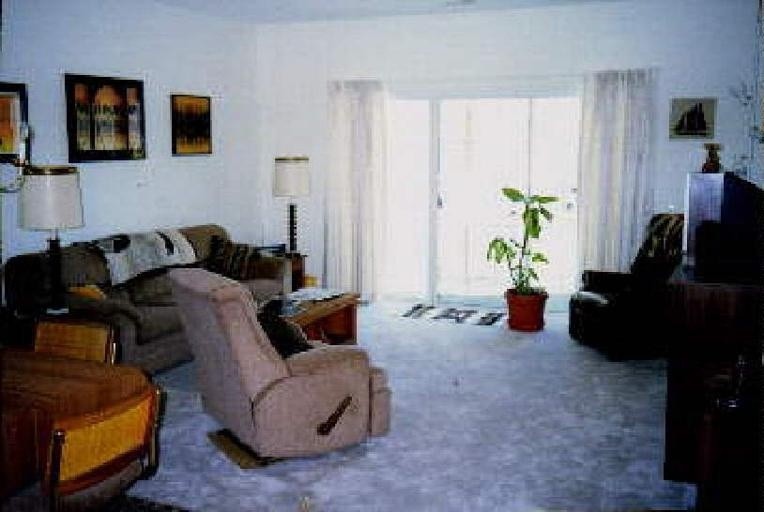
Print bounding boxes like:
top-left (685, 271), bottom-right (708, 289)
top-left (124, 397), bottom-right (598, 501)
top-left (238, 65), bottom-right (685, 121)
top-left (257, 302), bottom-right (311, 354)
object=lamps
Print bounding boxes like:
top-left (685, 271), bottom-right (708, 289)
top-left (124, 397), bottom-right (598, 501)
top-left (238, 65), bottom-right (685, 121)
top-left (19, 167), bottom-right (86, 315)
top-left (275, 157), bottom-right (309, 255)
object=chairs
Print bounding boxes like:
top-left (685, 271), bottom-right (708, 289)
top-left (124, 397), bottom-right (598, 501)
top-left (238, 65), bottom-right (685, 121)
top-left (167, 266), bottom-right (392, 468)
top-left (0, 321), bottom-right (166, 512)
top-left (568, 214), bottom-right (683, 362)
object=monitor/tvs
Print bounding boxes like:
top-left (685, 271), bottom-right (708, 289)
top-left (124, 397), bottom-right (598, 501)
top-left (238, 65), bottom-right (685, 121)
top-left (682, 171), bottom-right (764, 283)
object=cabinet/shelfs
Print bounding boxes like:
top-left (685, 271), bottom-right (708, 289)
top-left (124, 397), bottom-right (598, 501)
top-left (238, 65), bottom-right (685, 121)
top-left (663, 262), bottom-right (764, 512)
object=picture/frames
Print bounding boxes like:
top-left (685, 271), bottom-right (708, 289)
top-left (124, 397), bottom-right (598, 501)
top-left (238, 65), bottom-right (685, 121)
top-left (669, 95), bottom-right (719, 143)
top-left (0, 82), bottom-right (30, 163)
top-left (64, 73), bottom-right (145, 163)
top-left (170, 95), bottom-right (213, 155)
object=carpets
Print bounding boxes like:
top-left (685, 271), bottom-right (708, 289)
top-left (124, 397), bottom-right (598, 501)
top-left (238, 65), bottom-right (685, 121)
top-left (395, 302), bottom-right (509, 330)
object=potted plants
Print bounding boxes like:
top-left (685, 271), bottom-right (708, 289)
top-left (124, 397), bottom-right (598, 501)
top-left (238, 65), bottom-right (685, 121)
top-left (487, 188), bottom-right (559, 331)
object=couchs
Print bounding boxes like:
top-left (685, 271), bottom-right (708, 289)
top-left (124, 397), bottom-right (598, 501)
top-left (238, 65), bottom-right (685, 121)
top-left (0, 223), bottom-right (292, 374)
